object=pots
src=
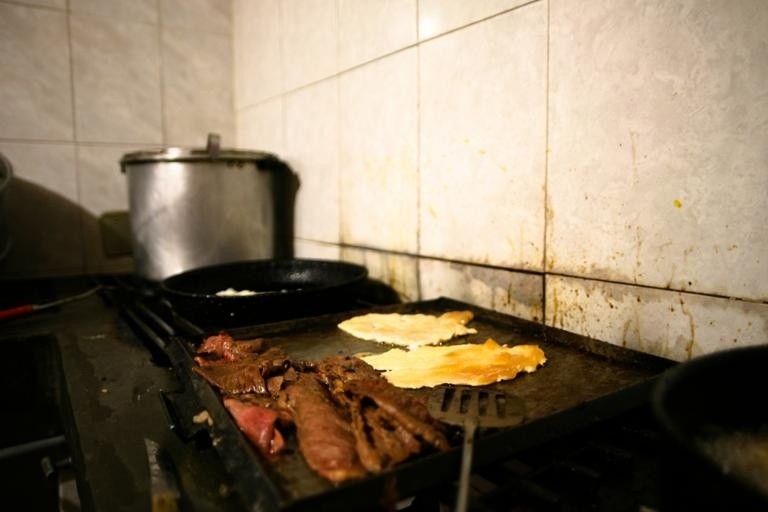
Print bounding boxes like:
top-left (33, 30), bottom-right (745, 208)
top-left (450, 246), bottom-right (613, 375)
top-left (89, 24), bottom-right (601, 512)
top-left (1, 260), bottom-right (367, 325)
top-left (119, 133), bottom-right (277, 285)
